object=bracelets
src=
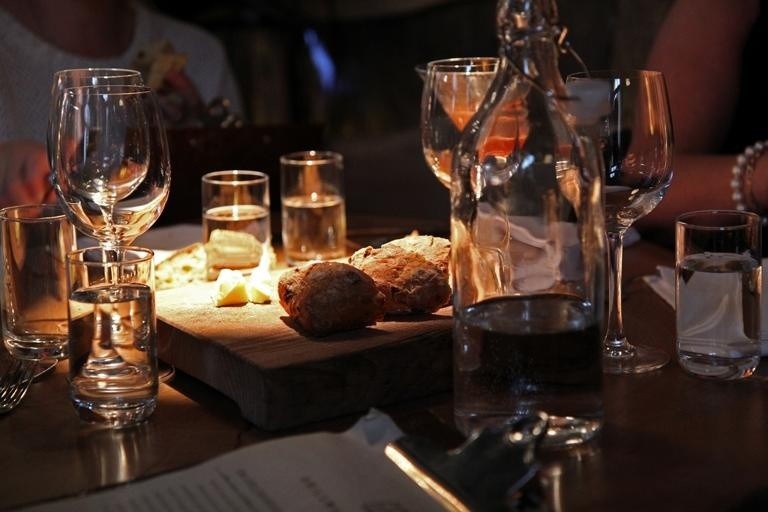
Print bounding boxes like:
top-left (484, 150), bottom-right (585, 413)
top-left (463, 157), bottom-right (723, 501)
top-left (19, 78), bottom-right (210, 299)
top-left (730, 143), bottom-right (768, 225)
top-left (741, 144), bottom-right (768, 215)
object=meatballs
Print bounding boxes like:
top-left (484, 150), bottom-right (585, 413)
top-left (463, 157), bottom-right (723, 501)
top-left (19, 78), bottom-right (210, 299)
top-left (276, 233), bottom-right (452, 339)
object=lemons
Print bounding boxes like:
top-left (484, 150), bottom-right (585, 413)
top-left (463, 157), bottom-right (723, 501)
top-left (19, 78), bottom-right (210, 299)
top-left (247, 280), bottom-right (275, 303)
top-left (212, 267), bottom-right (249, 306)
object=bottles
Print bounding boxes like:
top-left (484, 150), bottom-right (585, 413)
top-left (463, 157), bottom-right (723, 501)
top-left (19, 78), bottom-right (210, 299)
top-left (450, 0), bottom-right (608, 451)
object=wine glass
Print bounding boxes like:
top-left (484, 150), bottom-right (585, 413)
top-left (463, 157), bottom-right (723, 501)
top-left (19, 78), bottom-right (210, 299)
top-left (562, 69), bottom-right (675, 375)
top-left (49, 67), bottom-right (177, 385)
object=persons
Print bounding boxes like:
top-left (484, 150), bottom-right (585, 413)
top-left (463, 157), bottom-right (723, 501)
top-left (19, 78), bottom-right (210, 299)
top-left (2, 3), bottom-right (246, 234)
top-left (611, 1), bottom-right (767, 229)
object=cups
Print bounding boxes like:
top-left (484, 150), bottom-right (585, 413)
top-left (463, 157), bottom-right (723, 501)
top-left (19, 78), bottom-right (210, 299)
top-left (675, 210), bottom-right (762, 377)
top-left (0, 203), bottom-right (159, 430)
top-left (201, 149), bottom-right (348, 277)
top-left (419, 54), bottom-right (500, 190)
